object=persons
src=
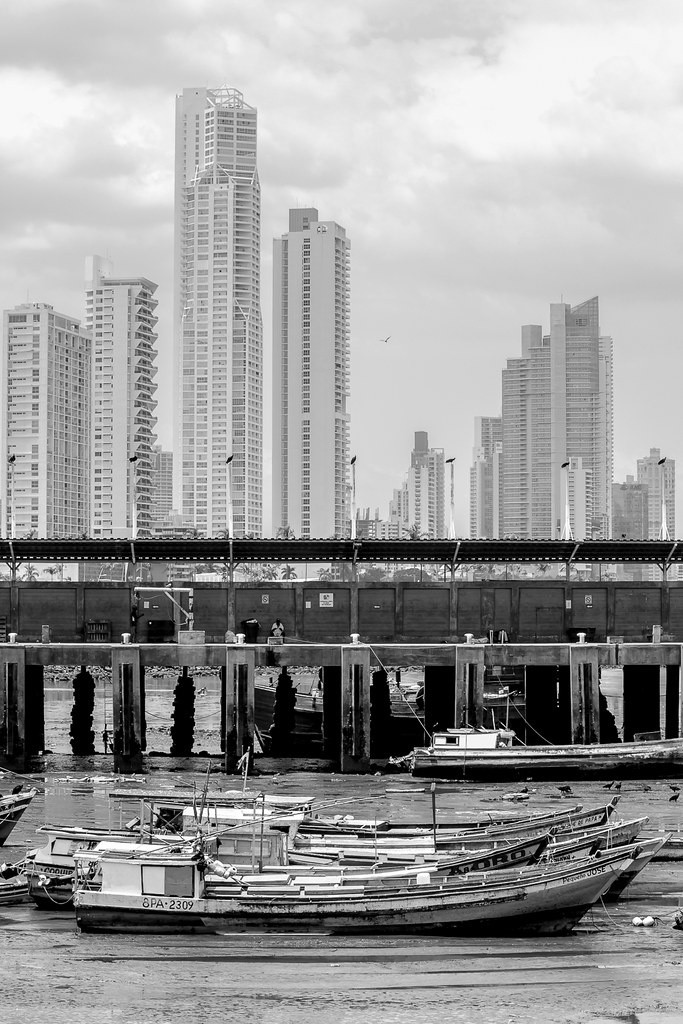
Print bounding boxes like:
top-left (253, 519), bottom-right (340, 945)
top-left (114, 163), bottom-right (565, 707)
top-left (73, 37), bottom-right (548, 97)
top-left (131, 605), bottom-right (144, 642)
top-left (271, 618), bottom-right (285, 643)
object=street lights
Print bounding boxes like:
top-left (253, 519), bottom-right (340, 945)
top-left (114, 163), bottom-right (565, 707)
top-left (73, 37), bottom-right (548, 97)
top-left (561, 463), bottom-right (570, 541)
top-left (128, 456), bottom-right (139, 534)
top-left (8, 453), bottom-right (17, 539)
top-left (658, 456), bottom-right (668, 541)
top-left (350, 454), bottom-right (357, 539)
top-left (445, 457), bottom-right (454, 538)
top-left (224, 455), bottom-right (235, 539)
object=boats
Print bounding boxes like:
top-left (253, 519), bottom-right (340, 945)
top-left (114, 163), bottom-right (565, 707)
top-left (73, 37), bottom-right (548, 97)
top-left (252, 669), bottom-right (524, 744)
top-left (23, 786), bottom-right (671, 938)
top-left (405, 726), bottom-right (683, 783)
top-left (0, 789), bottom-right (38, 847)
